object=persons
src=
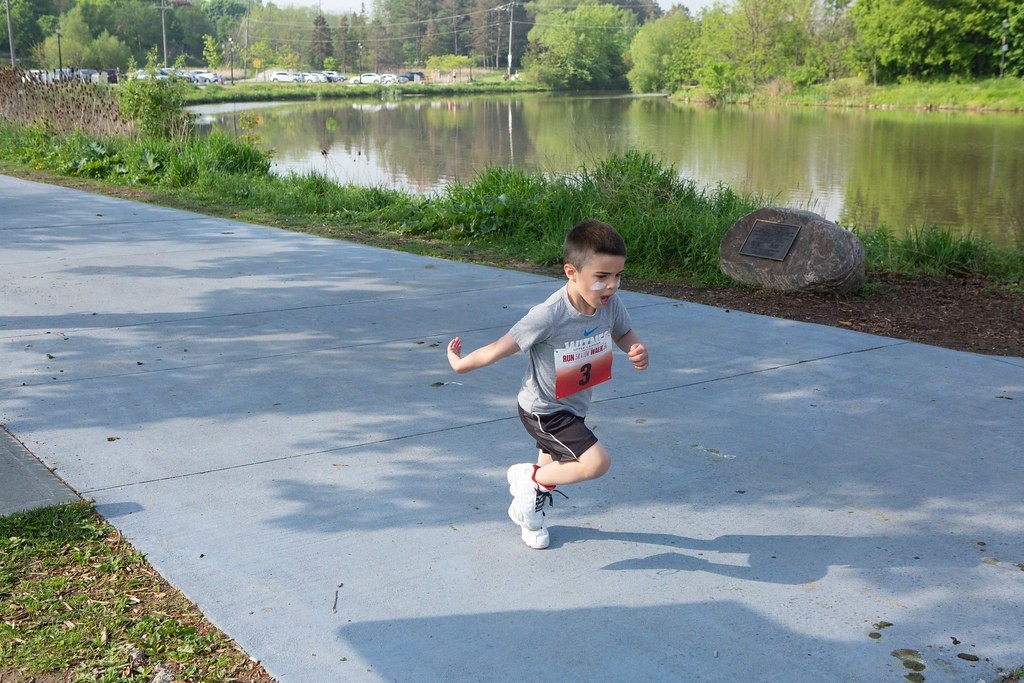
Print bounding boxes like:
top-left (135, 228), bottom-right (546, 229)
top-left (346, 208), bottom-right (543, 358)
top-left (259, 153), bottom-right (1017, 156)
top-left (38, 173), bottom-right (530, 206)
top-left (448, 221), bottom-right (650, 549)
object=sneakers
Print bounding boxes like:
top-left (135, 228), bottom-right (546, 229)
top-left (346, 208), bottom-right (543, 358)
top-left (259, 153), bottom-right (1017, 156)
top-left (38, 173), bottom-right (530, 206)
top-left (508, 498), bottom-right (550, 549)
top-left (507, 463), bottom-right (568, 531)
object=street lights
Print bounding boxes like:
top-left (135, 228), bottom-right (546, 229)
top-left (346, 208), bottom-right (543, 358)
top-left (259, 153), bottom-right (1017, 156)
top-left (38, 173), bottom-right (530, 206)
top-left (228, 37), bottom-right (234, 85)
top-left (55, 24), bottom-right (64, 84)
top-left (357, 42), bottom-right (364, 84)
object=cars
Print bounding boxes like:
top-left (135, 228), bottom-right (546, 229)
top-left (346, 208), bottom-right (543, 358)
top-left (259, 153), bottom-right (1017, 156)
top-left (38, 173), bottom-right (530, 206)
top-left (270, 70), bottom-right (347, 84)
top-left (19, 68), bottom-right (225, 86)
top-left (381, 71), bottom-right (425, 84)
top-left (350, 72), bottom-right (382, 84)
